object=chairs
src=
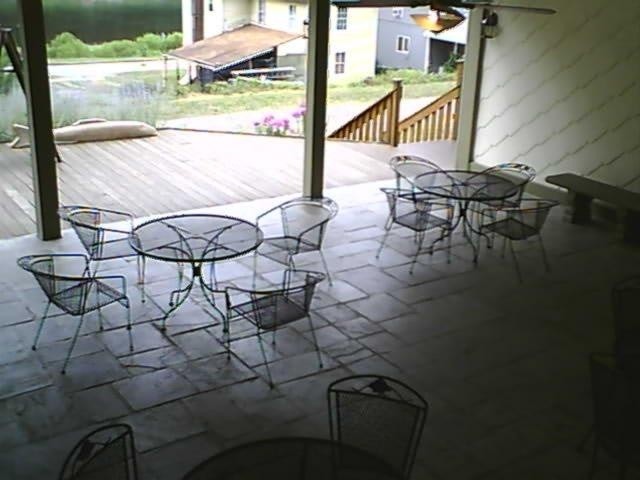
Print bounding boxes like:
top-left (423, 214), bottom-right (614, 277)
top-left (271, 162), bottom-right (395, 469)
top-left (16, 253), bottom-right (134, 375)
top-left (224, 268), bottom-right (328, 390)
top-left (374, 188), bottom-right (455, 275)
top-left (456, 163), bottom-right (536, 248)
top-left (254, 195), bottom-right (340, 291)
top-left (473, 198), bottom-right (560, 284)
top-left (326, 374), bottom-right (430, 478)
top-left (58, 422), bottom-right (138, 479)
top-left (387, 155), bottom-right (456, 240)
top-left (57, 204), bottom-right (148, 303)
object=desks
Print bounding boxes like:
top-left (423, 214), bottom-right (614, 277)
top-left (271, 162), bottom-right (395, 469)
top-left (184, 434), bottom-right (402, 478)
top-left (413, 170), bottom-right (519, 263)
top-left (129, 214), bottom-right (263, 335)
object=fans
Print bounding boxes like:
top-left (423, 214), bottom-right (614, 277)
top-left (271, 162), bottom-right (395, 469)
top-left (332, 0), bottom-right (560, 19)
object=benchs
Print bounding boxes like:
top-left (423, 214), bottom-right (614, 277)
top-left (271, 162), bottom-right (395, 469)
top-left (545, 172), bottom-right (639, 243)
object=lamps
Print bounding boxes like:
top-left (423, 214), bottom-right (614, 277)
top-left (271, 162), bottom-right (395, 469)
top-left (481, 10), bottom-right (499, 42)
top-left (408, 7), bottom-right (466, 37)
